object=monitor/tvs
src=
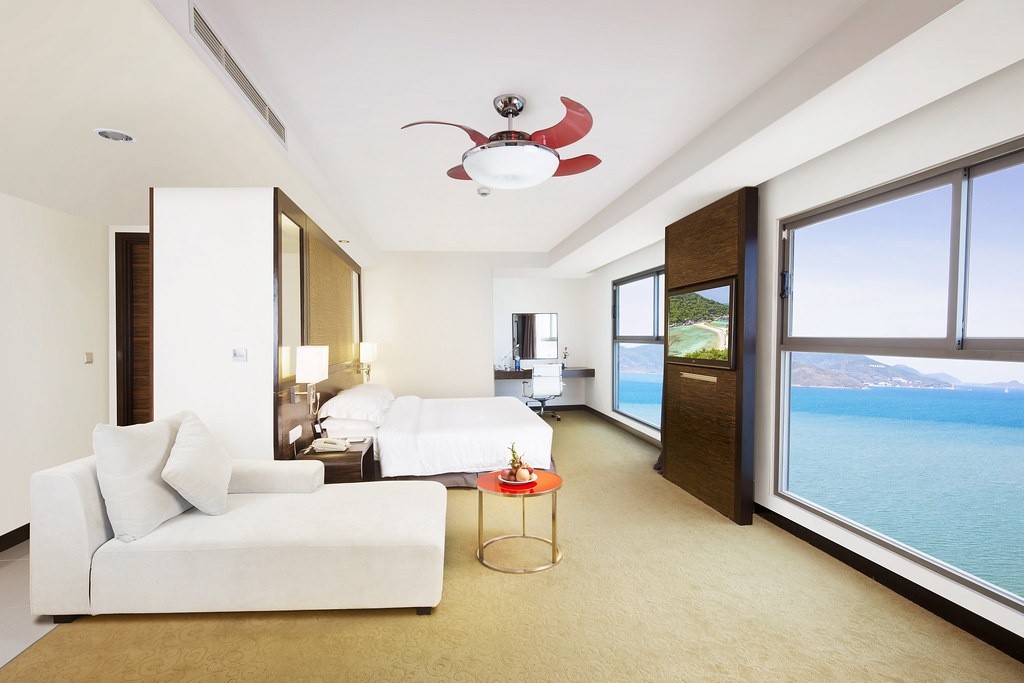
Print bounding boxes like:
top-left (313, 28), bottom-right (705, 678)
top-left (666, 278), bottom-right (736, 371)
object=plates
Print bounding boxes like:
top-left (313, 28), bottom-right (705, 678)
top-left (498, 474), bottom-right (537, 484)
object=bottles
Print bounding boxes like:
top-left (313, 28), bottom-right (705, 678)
top-left (515, 357), bottom-right (520, 371)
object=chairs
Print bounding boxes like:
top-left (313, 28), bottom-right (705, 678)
top-left (523, 364), bottom-right (565, 421)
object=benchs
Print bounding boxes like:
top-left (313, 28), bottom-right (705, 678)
top-left (28, 456), bottom-right (446, 615)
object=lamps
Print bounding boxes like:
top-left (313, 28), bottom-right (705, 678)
top-left (291, 345), bottom-right (328, 415)
top-left (357, 341), bottom-right (377, 380)
top-left (399, 95), bottom-right (601, 192)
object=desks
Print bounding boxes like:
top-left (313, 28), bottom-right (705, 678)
top-left (494, 367), bottom-right (595, 379)
top-left (474, 468), bottom-right (563, 574)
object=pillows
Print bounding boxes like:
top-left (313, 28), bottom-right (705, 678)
top-left (317, 383), bottom-right (392, 422)
top-left (92, 411), bottom-right (193, 541)
top-left (160, 413), bottom-right (234, 515)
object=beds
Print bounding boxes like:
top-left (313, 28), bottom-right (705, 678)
top-left (313, 396), bottom-right (556, 488)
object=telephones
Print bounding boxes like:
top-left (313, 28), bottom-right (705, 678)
top-left (313, 438), bottom-right (350, 453)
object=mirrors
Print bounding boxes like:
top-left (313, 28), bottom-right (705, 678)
top-left (512, 313), bottom-right (558, 360)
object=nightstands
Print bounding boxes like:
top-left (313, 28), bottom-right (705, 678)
top-left (295, 436), bottom-right (374, 483)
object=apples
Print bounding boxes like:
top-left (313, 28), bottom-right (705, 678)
top-left (501, 468), bottom-right (531, 482)
